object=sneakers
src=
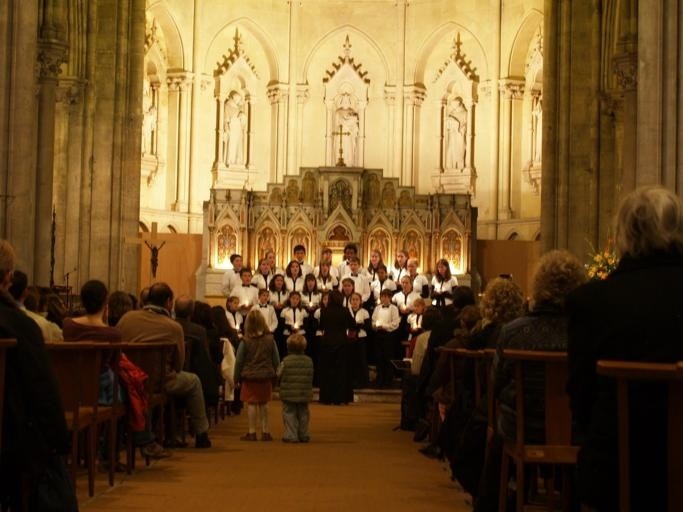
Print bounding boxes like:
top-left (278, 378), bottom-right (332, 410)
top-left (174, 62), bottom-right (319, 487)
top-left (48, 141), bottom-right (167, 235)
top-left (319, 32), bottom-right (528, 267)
top-left (240, 433), bottom-right (257, 440)
top-left (261, 432), bottom-right (273, 442)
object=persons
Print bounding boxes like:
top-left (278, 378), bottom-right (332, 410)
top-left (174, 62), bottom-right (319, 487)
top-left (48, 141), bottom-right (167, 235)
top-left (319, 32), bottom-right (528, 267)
top-left (172, 294), bottom-right (244, 419)
top-left (445, 98), bottom-right (468, 170)
top-left (269, 173), bottom-right (416, 209)
top-left (141, 104), bottom-right (155, 155)
top-left (488, 248), bottom-right (592, 477)
top-left (221, 244), bottom-right (458, 391)
top-left (2, 240), bottom-right (78, 512)
top-left (439, 276), bottom-right (525, 491)
top-left (108, 284), bottom-right (212, 448)
top-left (319, 289), bottom-right (358, 404)
top-left (563, 183), bottom-right (681, 511)
top-left (330, 93), bottom-right (358, 166)
top-left (276, 333), bottom-right (314, 443)
top-left (234, 309), bottom-right (280, 442)
top-left (9, 270), bottom-right (170, 472)
top-left (222, 92), bottom-right (246, 166)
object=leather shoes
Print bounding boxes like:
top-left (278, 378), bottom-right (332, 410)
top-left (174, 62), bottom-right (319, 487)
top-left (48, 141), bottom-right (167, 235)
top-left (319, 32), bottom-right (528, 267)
top-left (98, 459), bottom-right (128, 473)
top-left (195, 433), bottom-right (211, 448)
top-left (413, 419), bottom-right (431, 441)
top-left (162, 438), bottom-right (189, 449)
top-left (418, 446), bottom-right (443, 460)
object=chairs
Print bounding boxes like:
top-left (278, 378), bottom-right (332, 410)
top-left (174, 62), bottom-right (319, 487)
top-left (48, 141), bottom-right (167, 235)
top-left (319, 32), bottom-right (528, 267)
top-left (0, 336), bottom-right (227, 511)
top-left (394, 345), bottom-right (682, 512)
